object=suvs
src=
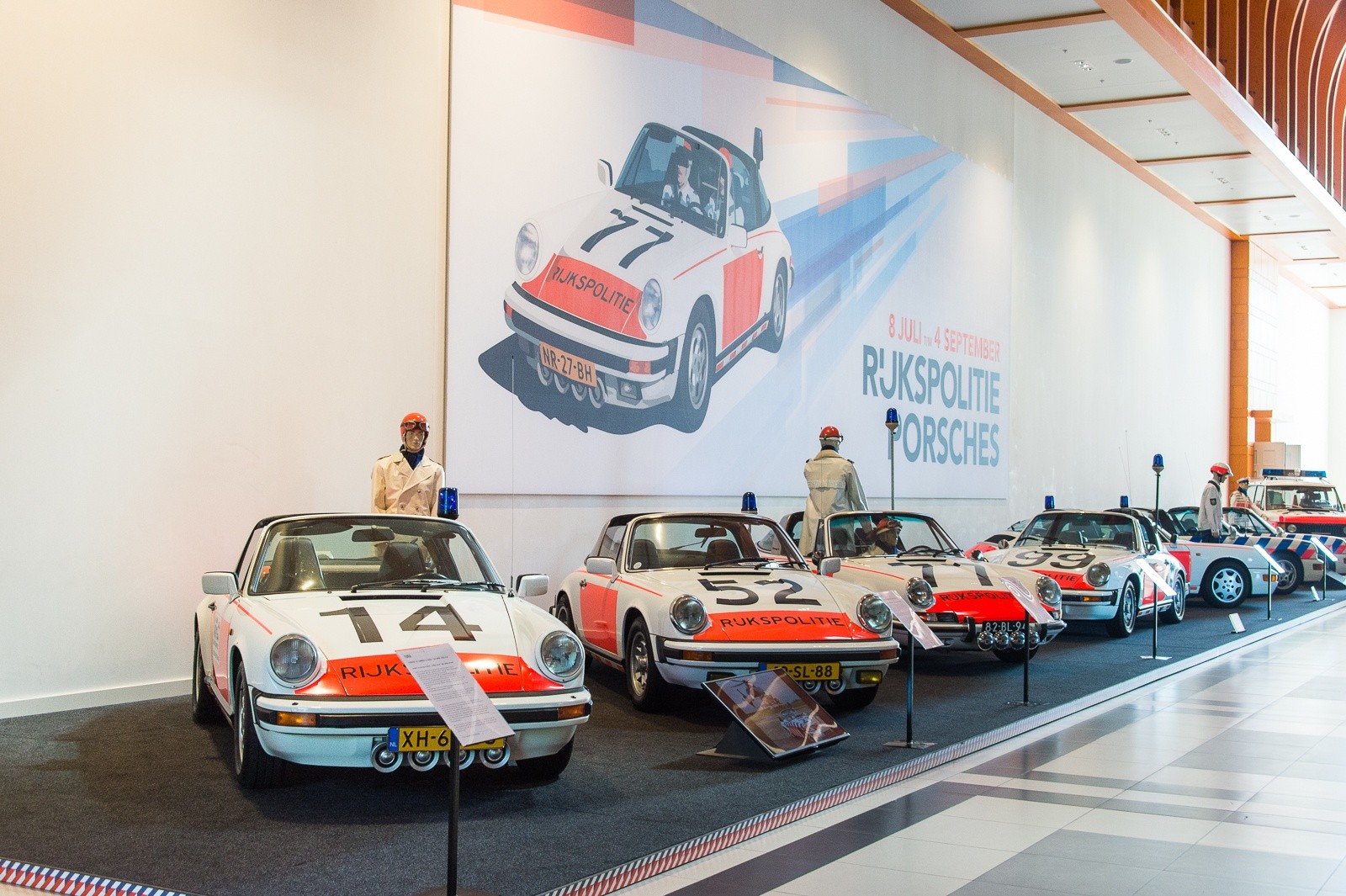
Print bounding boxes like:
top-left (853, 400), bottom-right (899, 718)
top-left (1245, 467), bottom-right (1346, 539)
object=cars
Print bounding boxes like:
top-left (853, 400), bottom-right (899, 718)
top-left (964, 518), bottom-right (1034, 559)
top-left (1101, 494), bottom-right (1346, 610)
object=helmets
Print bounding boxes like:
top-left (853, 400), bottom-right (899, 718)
top-left (714, 148), bottom-right (733, 169)
top-left (819, 426), bottom-right (840, 440)
top-left (876, 518), bottom-right (902, 536)
top-left (1211, 462), bottom-right (1233, 476)
top-left (401, 413), bottom-right (429, 444)
top-left (675, 140), bottom-right (692, 166)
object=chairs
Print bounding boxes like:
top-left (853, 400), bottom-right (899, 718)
top-left (378, 542), bottom-right (426, 582)
top-left (1301, 494), bottom-right (1321, 508)
top-left (1058, 530), bottom-right (1083, 545)
top-left (265, 538), bottom-right (327, 592)
top-left (1183, 519), bottom-right (1196, 531)
top-left (1113, 531), bottom-right (1133, 548)
top-left (1268, 491), bottom-right (1283, 505)
top-left (817, 526), bottom-right (880, 558)
top-left (704, 539), bottom-right (741, 566)
top-left (631, 539), bottom-right (661, 570)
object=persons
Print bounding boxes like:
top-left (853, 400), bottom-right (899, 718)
top-left (1198, 462), bottom-right (1234, 543)
top-left (704, 170), bottom-right (744, 226)
top-left (858, 518), bottom-right (904, 556)
top-left (661, 152), bottom-right (702, 213)
top-left (798, 426), bottom-right (873, 556)
top-left (372, 412), bottom-right (444, 561)
top-left (1230, 477), bottom-right (1269, 527)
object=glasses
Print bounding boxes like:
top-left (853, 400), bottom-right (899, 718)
top-left (887, 520), bottom-right (902, 529)
top-left (839, 433), bottom-right (843, 442)
top-left (403, 420), bottom-right (429, 431)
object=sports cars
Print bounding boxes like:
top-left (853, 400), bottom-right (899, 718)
top-left (755, 511), bottom-right (1068, 666)
top-left (549, 492), bottom-right (901, 715)
top-left (981, 496), bottom-right (1191, 639)
top-left (192, 487), bottom-right (593, 792)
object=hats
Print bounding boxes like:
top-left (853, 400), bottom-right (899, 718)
top-left (1236, 477), bottom-right (1249, 484)
